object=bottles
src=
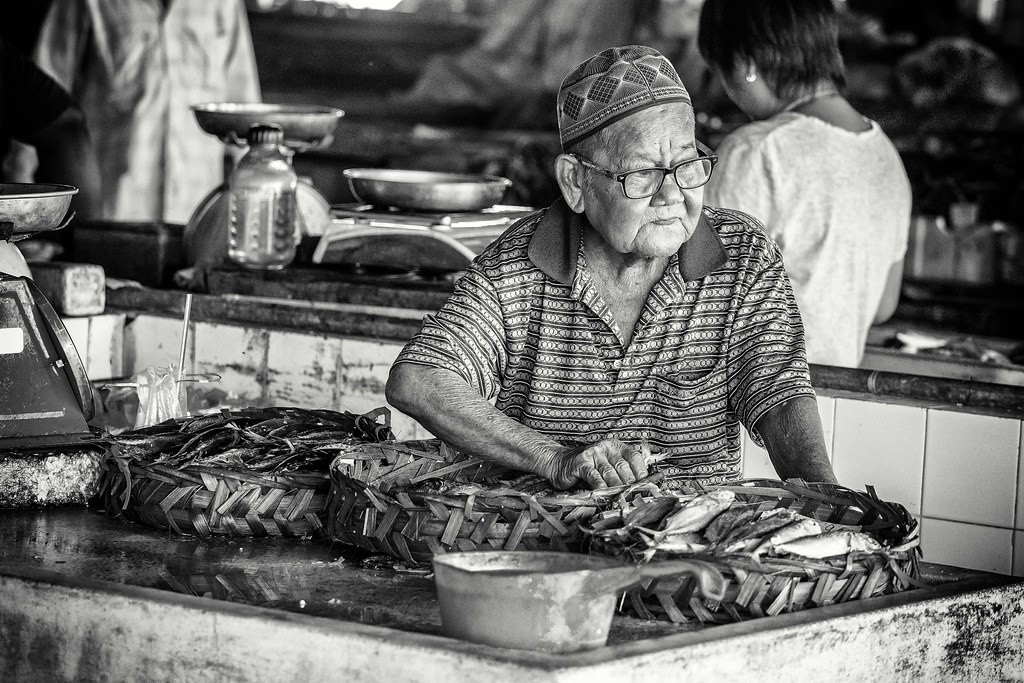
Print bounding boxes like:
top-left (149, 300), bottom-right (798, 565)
top-left (228, 123), bottom-right (296, 268)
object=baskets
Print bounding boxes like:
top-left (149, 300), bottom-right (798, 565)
top-left (94, 407), bottom-right (925, 626)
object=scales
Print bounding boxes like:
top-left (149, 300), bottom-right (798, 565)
top-left (1, 183), bottom-right (112, 450)
top-left (179, 100), bottom-right (346, 269)
top-left (314, 199), bottom-right (537, 275)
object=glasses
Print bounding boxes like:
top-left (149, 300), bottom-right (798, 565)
top-left (568, 139), bottom-right (719, 199)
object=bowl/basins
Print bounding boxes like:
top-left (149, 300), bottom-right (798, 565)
top-left (435, 551), bottom-right (622, 651)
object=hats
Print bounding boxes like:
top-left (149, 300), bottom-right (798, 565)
top-left (557, 46), bottom-right (693, 149)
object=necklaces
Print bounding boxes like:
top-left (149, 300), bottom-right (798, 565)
top-left (783, 90), bottom-right (837, 112)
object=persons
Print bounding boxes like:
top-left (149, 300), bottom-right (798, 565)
top-left (0, 0), bottom-right (69, 186)
top-left (691, 0), bottom-right (917, 372)
top-left (383, 39), bottom-right (839, 500)
top-left (2, 0), bottom-right (268, 239)
